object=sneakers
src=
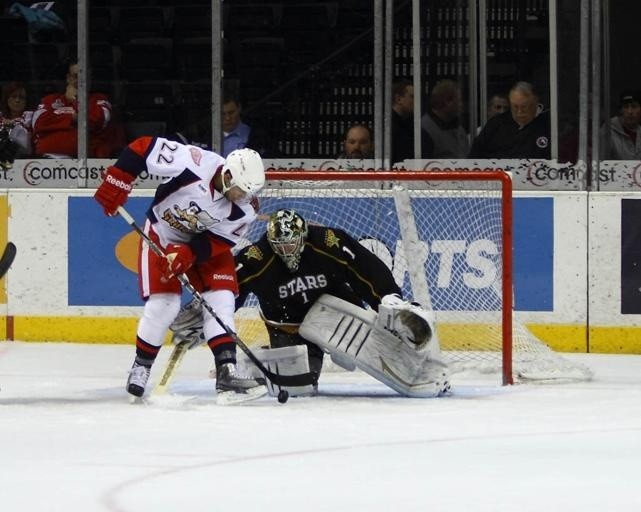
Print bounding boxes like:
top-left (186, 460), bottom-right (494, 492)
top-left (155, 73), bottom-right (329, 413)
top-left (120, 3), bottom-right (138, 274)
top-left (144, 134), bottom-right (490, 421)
top-left (124, 360), bottom-right (153, 398)
top-left (213, 362), bottom-right (266, 391)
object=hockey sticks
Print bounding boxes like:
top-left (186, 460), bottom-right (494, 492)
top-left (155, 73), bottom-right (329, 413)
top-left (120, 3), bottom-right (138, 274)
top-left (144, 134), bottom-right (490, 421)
top-left (148, 341), bottom-right (199, 405)
top-left (117, 206), bottom-right (319, 387)
top-left (0, 242), bottom-right (17, 280)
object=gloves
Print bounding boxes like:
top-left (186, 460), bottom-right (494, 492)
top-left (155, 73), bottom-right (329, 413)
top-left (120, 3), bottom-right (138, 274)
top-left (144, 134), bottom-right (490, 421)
top-left (92, 164), bottom-right (137, 218)
top-left (157, 242), bottom-right (198, 280)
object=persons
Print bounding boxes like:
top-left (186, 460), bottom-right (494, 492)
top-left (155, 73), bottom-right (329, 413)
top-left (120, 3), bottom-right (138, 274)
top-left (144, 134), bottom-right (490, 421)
top-left (221, 93), bottom-right (266, 158)
top-left (94, 134), bottom-right (267, 400)
top-left (0, 62), bottom-right (113, 161)
top-left (390, 77), bottom-right (552, 160)
top-left (599, 92), bottom-right (640, 161)
top-left (172, 209), bottom-right (450, 400)
top-left (345, 124), bottom-right (375, 159)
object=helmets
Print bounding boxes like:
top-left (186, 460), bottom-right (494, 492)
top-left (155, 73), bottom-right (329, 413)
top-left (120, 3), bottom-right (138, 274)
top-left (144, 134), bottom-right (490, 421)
top-left (220, 147), bottom-right (265, 205)
top-left (266, 207), bottom-right (308, 270)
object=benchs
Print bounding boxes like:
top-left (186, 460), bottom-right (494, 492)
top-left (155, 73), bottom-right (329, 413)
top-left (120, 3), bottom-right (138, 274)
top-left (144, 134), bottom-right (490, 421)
top-left (0, 0), bottom-right (337, 139)
